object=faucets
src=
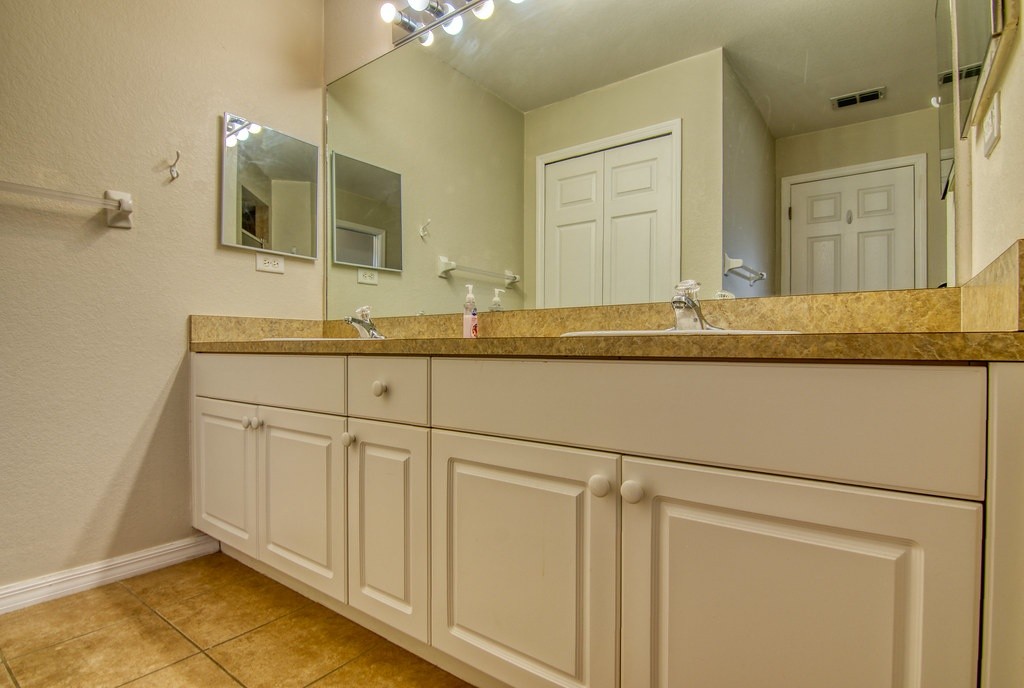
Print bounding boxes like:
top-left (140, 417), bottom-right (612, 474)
top-left (344, 306), bottom-right (385, 337)
top-left (664, 279), bottom-right (725, 330)
top-left (713, 289), bottom-right (736, 299)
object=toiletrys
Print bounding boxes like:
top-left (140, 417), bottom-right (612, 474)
top-left (463, 285), bottom-right (478, 337)
top-left (489, 289), bottom-right (505, 311)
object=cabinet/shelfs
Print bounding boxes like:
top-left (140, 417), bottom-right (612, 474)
top-left (545, 132), bottom-right (672, 309)
top-left (431, 357), bottom-right (986, 688)
top-left (342, 356), bottom-right (431, 648)
top-left (239, 181), bottom-right (271, 249)
top-left (192, 354), bottom-right (347, 606)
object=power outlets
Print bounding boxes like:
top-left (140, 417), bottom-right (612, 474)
top-left (357, 268), bottom-right (379, 285)
top-left (256, 252), bottom-right (285, 274)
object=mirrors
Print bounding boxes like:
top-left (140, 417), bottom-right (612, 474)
top-left (324, 0), bottom-right (953, 319)
top-left (956, 0), bottom-right (992, 139)
top-left (221, 112), bottom-right (321, 260)
top-left (935, 0), bottom-right (953, 199)
top-left (332, 150), bottom-right (404, 273)
